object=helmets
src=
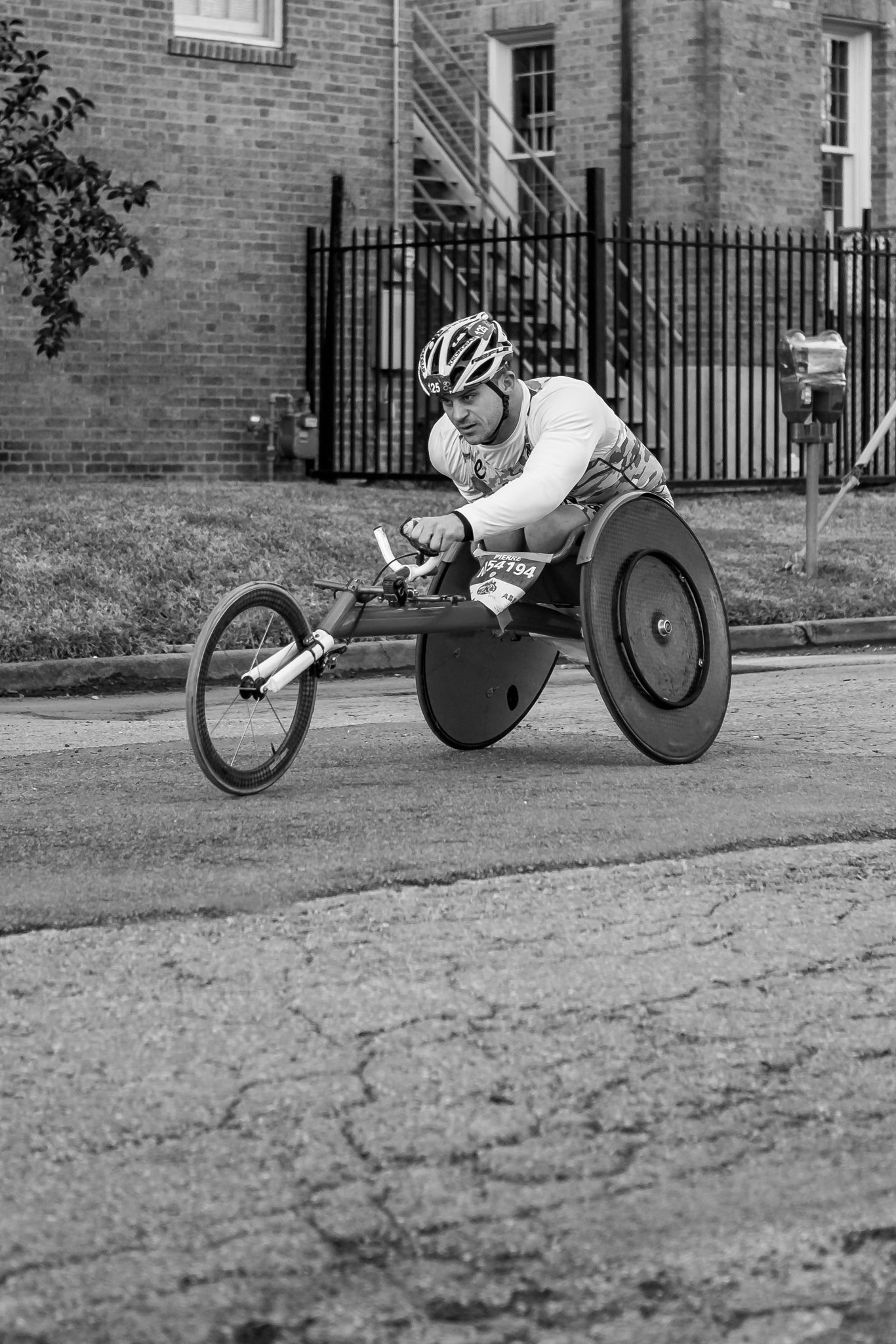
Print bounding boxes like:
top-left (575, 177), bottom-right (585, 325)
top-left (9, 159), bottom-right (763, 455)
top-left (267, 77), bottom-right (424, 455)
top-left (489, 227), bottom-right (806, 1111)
top-left (418, 311), bottom-right (514, 398)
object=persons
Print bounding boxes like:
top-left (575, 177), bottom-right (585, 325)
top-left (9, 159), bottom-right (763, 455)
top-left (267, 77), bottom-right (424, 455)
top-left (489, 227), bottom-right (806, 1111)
top-left (411, 312), bottom-right (675, 554)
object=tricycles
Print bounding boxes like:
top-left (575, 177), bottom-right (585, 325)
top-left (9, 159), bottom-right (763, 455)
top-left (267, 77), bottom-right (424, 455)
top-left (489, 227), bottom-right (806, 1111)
top-left (185, 489), bottom-right (733, 797)
